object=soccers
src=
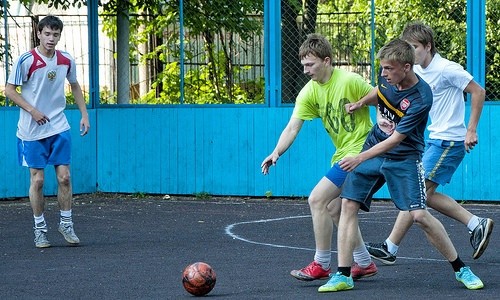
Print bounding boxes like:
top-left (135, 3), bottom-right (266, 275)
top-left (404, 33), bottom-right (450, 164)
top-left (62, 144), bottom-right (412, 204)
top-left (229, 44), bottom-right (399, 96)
top-left (182, 262), bottom-right (216, 296)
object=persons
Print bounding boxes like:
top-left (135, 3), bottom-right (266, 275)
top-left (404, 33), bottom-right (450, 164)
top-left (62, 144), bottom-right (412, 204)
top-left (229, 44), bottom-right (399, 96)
top-left (260, 36), bottom-right (395, 282)
top-left (317, 38), bottom-right (484, 293)
top-left (363, 24), bottom-right (493, 266)
top-left (5, 16), bottom-right (90, 248)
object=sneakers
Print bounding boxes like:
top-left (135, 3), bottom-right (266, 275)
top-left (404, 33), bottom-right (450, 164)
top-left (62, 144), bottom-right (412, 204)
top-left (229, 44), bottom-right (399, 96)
top-left (34, 229), bottom-right (50, 247)
top-left (455, 266), bottom-right (484, 289)
top-left (351, 263), bottom-right (379, 280)
top-left (58, 222), bottom-right (79, 243)
top-left (364, 241), bottom-right (396, 265)
top-left (318, 271), bottom-right (354, 292)
top-left (469, 217), bottom-right (494, 260)
top-left (290, 261), bottom-right (331, 281)
